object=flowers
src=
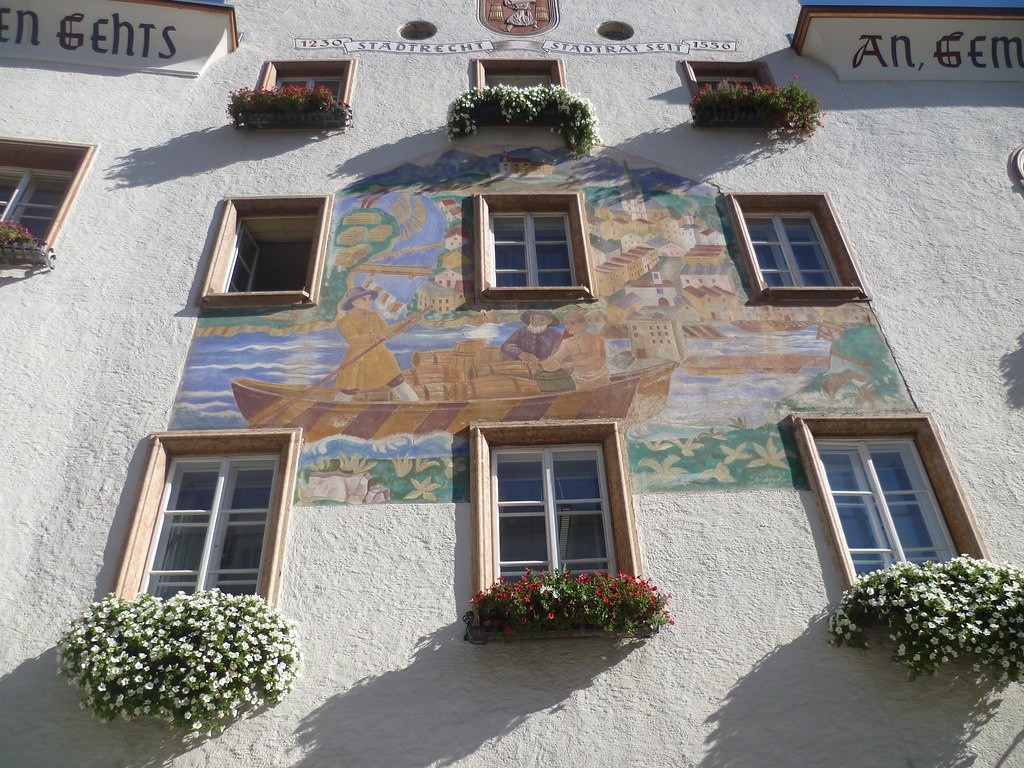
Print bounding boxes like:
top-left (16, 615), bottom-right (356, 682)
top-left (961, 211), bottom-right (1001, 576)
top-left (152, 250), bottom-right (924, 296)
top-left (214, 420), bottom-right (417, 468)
top-left (55, 588), bottom-right (304, 739)
top-left (826, 553), bottom-right (1024, 692)
top-left (445, 84), bottom-right (602, 161)
top-left (469, 564), bottom-right (674, 639)
top-left (225, 84), bottom-right (351, 130)
top-left (689, 74), bottom-right (826, 139)
top-left (0, 214), bottom-right (35, 243)
